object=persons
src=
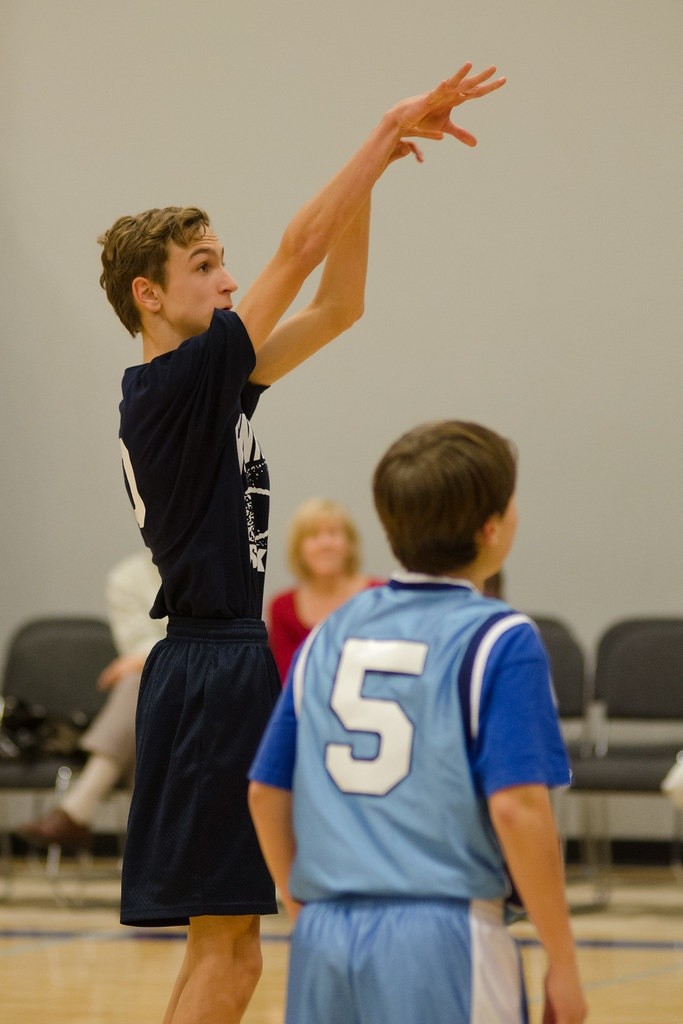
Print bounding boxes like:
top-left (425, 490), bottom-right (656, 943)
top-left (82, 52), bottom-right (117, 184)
top-left (19, 549), bottom-right (168, 844)
top-left (483, 570), bottom-right (540, 940)
top-left (248, 421), bottom-right (588, 1024)
top-left (268, 502), bottom-right (389, 686)
top-left (100, 60), bottom-right (506, 1024)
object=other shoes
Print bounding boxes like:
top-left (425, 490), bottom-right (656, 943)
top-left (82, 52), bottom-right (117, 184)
top-left (12, 806), bottom-right (84, 857)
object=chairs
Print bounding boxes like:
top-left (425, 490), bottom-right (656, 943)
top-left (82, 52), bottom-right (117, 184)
top-left (1, 618), bottom-right (128, 875)
top-left (531, 612), bottom-right (683, 914)
